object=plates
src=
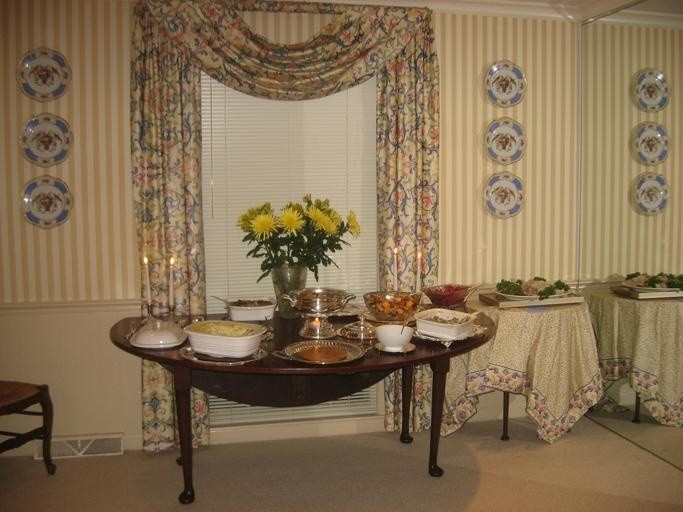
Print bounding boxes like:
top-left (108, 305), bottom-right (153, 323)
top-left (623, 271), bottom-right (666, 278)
top-left (14, 46), bottom-right (74, 104)
top-left (636, 67), bottom-right (668, 111)
top-left (303, 301), bottom-right (365, 318)
top-left (483, 171), bottom-right (525, 220)
top-left (19, 173), bottom-right (73, 229)
top-left (494, 290), bottom-right (571, 300)
top-left (16, 111), bottom-right (75, 169)
top-left (630, 123), bottom-right (671, 168)
top-left (486, 118), bottom-right (527, 164)
top-left (179, 345), bottom-right (265, 366)
top-left (621, 280), bottom-right (679, 293)
top-left (364, 314), bottom-right (486, 353)
top-left (273, 340), bottom-right (367, 365)
top-left (484, 60), bottom-right (526, 107)
top-left (634, 173), bottom-right (671, 215)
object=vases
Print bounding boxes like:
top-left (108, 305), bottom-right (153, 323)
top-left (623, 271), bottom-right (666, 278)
top-left (270, 264), bottom-right (307, 320)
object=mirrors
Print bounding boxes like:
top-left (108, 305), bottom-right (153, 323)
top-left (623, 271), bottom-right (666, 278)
top-left (577, 2), bottom-right (682, 470)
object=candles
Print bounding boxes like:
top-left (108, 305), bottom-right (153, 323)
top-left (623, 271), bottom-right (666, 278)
top-left (143, 256), bottom-right (151, 303)
top-left (169, 257), bottom-right (174, 305)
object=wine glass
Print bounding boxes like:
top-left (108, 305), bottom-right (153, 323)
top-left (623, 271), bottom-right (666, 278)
top-left (282, 288), bottom-right (356, 338)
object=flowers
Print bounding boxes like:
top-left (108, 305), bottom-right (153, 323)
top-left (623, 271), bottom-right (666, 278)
top-left (238, 194), bottom-right (361, 311)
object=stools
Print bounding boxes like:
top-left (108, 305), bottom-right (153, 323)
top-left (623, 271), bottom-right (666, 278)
top-left (1, 379), bottom-right (56, 474)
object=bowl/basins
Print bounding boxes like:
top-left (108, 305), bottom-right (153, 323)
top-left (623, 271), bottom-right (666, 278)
top-left (363, 291), bottom-right (421, 321)
top-left (422, 284), bottom-right (475, 307)
